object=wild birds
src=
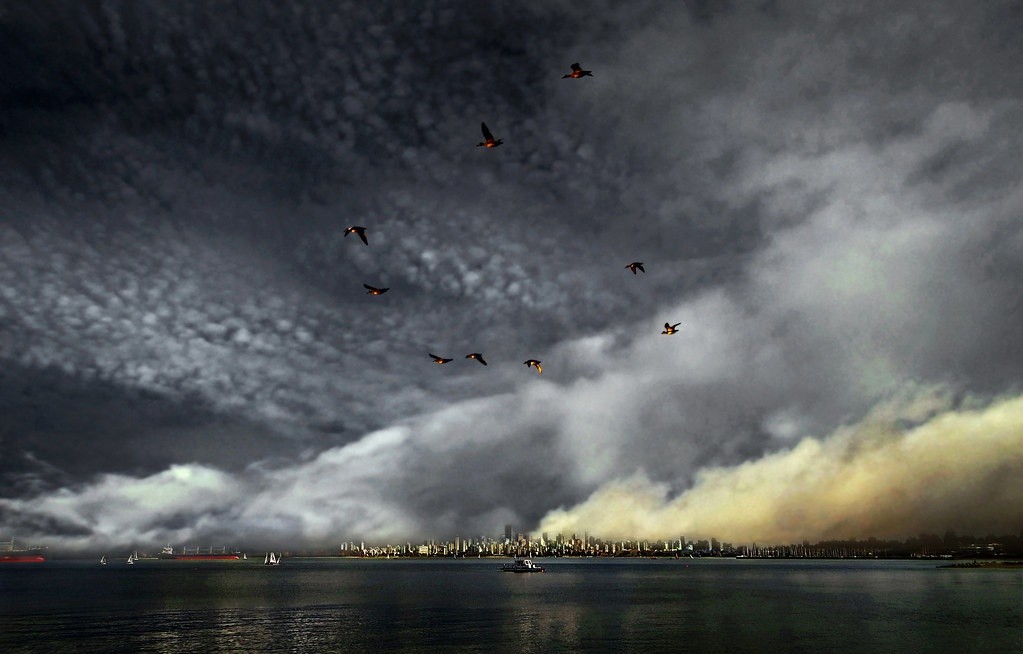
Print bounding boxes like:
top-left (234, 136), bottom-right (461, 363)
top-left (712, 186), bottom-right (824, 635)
top-left (561, 62), bottom-right (595, 79)
top-left (465, 353), bottom-right (487, 366)
top-left (522, 359), bottom-right (542, 376)
top-left (476, 122), bottom-right (504, 148)
top-left (364, 283), bottom-right (390, 294)
top-left (661, 322), bottom-right (681, 335)
top-left (623, 262), bottom-right (645, 275)
top-left (343, 227), bottom-right (369, 246)
top-left (429, 353), bottom-right (453, 364)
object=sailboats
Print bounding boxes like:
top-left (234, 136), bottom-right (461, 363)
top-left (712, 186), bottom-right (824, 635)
top-left (126, 551), bottom-right (139, 566)
top-left (263, 551), bottom-right (286, 567)
top-left (99, 555), bottom-right (107, 566)
top-left (243, 553), bottom-right (248, 560)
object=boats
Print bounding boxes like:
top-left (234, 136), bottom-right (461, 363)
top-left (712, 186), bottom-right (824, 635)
top-left (157, 542), bottom-right (242, 562)
top-left (498, 558), bottom-right (547, 573)
top-left (0, 537), bottom-right (49, 571)
